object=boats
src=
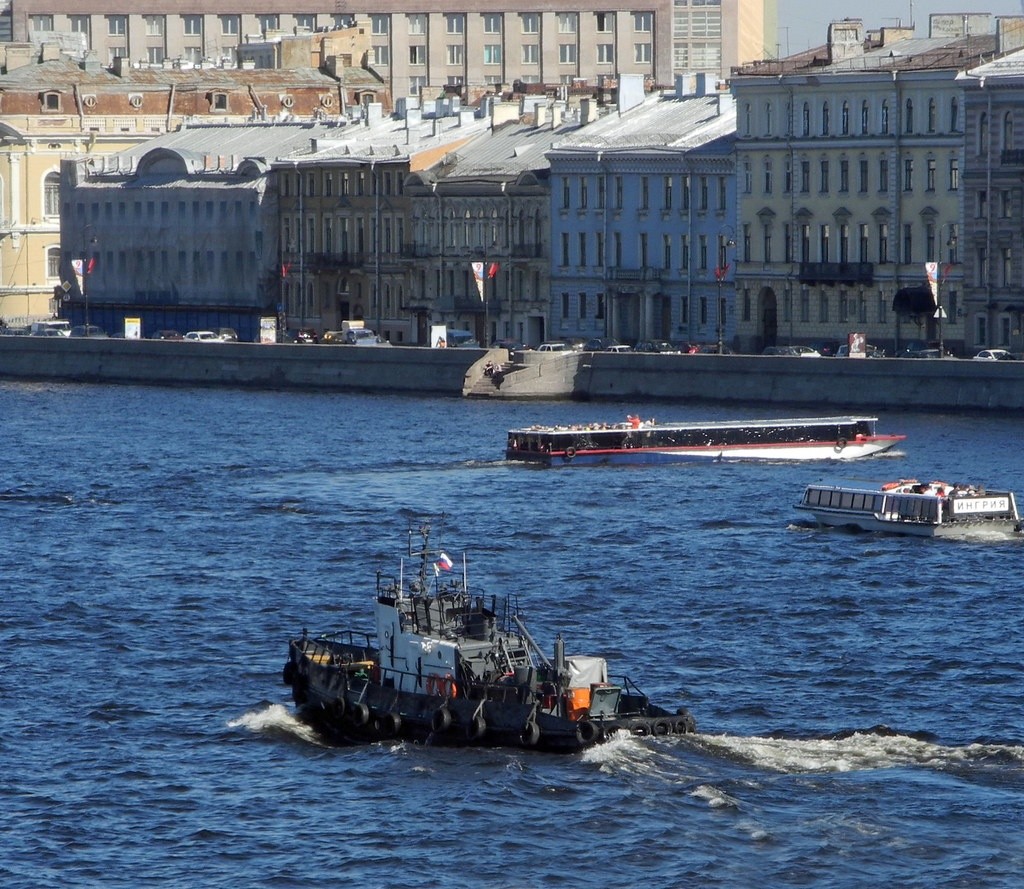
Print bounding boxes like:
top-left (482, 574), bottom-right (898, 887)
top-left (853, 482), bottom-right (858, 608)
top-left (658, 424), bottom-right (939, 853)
top-left (279, 522), bottom-right (694, 751)
top-left (792, 477), bottom-right (1024, 544)
top-left (507, 416), bottom-right (909, 469)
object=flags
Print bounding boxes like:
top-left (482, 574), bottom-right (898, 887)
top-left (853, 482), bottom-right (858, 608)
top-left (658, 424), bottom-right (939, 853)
top-left (437, 552), bottom-right (453, 571)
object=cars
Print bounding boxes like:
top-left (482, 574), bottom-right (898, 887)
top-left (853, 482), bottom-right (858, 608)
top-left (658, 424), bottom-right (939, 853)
top-left (28, 320), bottom-right (1019, 360)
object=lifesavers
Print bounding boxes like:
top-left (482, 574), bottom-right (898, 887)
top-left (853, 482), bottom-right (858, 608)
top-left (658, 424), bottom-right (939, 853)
top-left (332, 697), bottom-right (346, 718)
top-left (433, 706), bottom-right (451, 733)
top-left (371, 710), bottom-right (401, 736)
top-left (440, 674), bottom-right (457, 698)
top-left (603, 724), bottom-right (624, 739)
top-left (565, 446), bottom-right (576, 458)
top-left (653, 719), bottom-right (673, 736)
top-left (673, 719), bottom-right (688, 733)
top-left (426, 672), bottom-right (441, 696)
top-left (469, 715), bottom-right (486, 739)
top-left (519, 721), bottom-right (539, 747)
top-left (630, 721), bottom-right (652, 737)
top-left (350, 702), bottom-right (369, 726)
top-left (575, 720), bottom-right (599, 742)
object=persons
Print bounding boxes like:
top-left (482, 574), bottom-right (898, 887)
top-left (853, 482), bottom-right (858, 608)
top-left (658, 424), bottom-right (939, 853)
top-left (642, 418), bottom-right (654, 429)
top-left (629, 414), bottom-right (640, 429)
top-left (904, 482), bottom-right (986, 508)
top-left (483, 361), bottom-right (502, 376)
